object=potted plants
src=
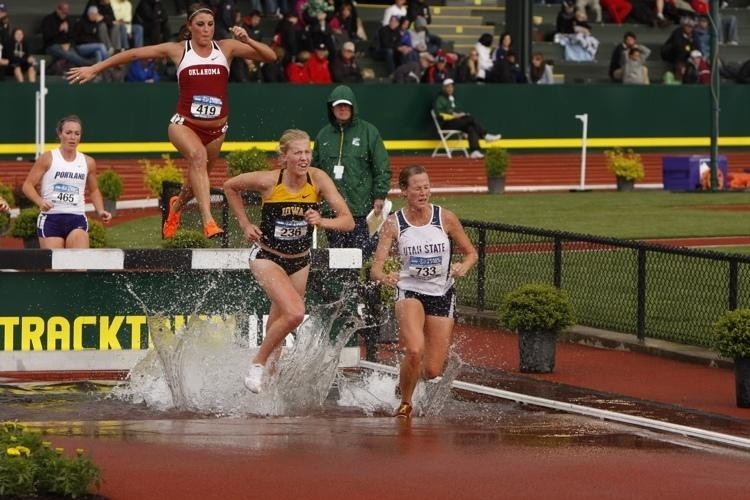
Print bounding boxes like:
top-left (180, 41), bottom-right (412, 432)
top-left (709, 305), bottom-right (749, 411)
top-left (93, 166), bottom-right (127, 219)
top-left (482, 143), bottom-right (512, 194)
top-left (599, 142), bottom-right (645, 193)
top-left (490, 280), bottom-right (581, 377)
top-left (356, 253), bottom-right (406, 347)
top-left (7, 204), bottom-right (43, 248)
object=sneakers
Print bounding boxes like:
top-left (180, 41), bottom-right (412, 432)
top-left (392, 401), bottom-right (415, 419)
top-left (160, 196), bottom-right (184, 240)
top-left (243, 362), bottom-right (265, 395)
top-left (470, 150), bottom-right (485, 159)
top-left (202, 220), bottom-right (226, 240)
top-left (394, 383), bottom-right (402, 400)
top-left (485, 133), bottom-right (502, 144)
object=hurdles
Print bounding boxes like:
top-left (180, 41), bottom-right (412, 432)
top-left (0, 249), bottom-right (362, 373)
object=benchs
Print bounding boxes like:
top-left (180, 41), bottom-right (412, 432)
top-left (0, 2), bottom-right (194, 83)
top-left (526, 0), bottom-right (749, 85)
top-left (211, 0), bottom-right (409, 82)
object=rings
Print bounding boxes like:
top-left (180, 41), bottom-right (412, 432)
top-left (76, 77), bottom-right (81, 81)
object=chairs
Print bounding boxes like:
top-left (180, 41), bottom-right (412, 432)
top-left (429, 109), bottom-right (472, 161)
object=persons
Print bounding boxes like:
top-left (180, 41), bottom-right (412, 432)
top-left (0, 1), bottom-right (750, 90)
top-left (0, 197), bottom-right (11, 213)
top-left (224, 127), bottom-right (356, 403)
top-left (369, 162), bottom-right (480, 419)
top-left (63, 1), bottom-right (277, 239)
top-left (22, 116), bottom-right (112, 250)
top-left (431, 73), bottom-right (504, 160)
top-left (312, 83), bottom-right (393, 364)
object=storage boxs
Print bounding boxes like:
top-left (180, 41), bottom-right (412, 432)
top-left (659, 154), bottom-right (730, 194)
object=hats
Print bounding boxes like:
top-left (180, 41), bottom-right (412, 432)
top-left (314, 40), bottom-right (329, 51)
top-left (331, 99), bottom-right (353, 107)
top-left (690, 50), bottom-right (702, 59)
top-left (434, 55), bottom-right (446, 63)
top-left (343, 42), bottom-right (356, 52)
top-left (442, 78), bottom-right (456, 85)
